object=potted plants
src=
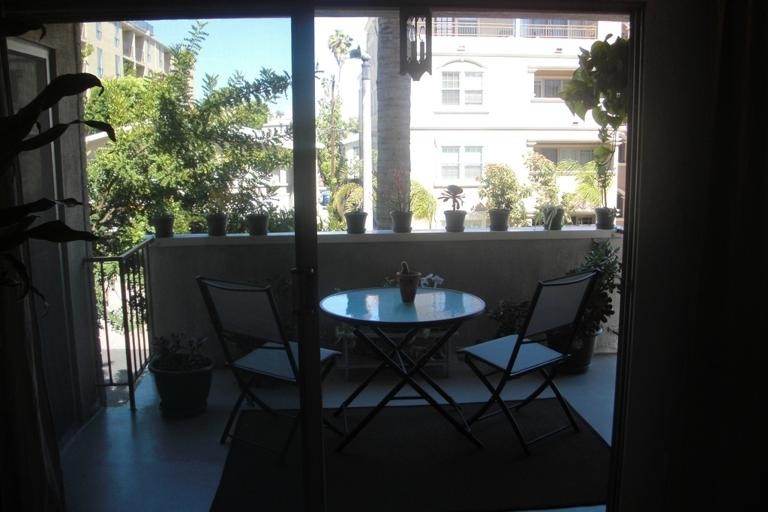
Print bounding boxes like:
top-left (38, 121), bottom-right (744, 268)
top-left (538, 201), bottom-right (569, 231)
top-left (247, 193), bottom-right (270, 236)
top-left (476, 163), bottom-right (517, 232)
top-left (345, 201), bottom-right (368, 235)
top-left (148, 183), bottom-right (175, 237)
top-left (397, 262), bottom-right (421, 306)
top-left (554, 241), bottom-right (621, 373)
top-left (390, 175), bottom-right (414, 232)
top-left (148, 337), bottom-right (212, 413)
top-left (204, 196), bottom-right (226, 237)
top-left (439, 185), bottom-right (467, 232)
top-left (593, 162), bottom-right (617, 230)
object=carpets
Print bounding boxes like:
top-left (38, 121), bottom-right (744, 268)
top-left (208, 396), bottom-right (611, 511)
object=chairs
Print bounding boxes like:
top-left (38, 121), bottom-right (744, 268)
top-left (456, 269), bottom-right (598, 455)
top-left (194, 275), bottom-right (345, 467)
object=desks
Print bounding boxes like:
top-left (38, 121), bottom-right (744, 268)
top-left (319, 286), bottom-right (487, 455)
